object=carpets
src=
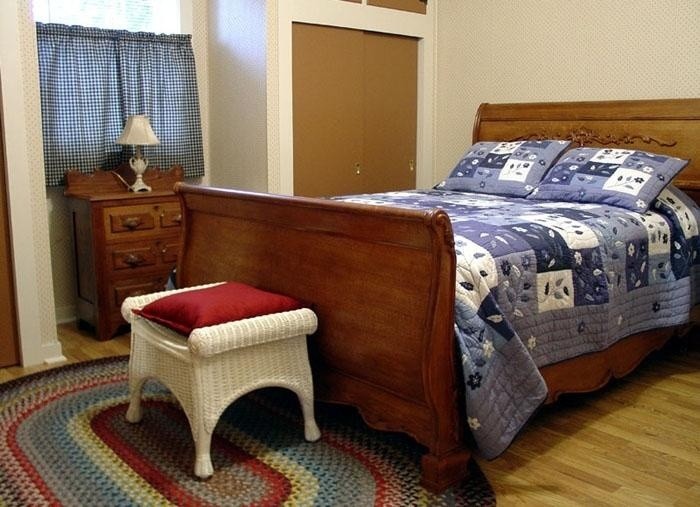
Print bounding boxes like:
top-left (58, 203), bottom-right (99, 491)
top-left (0, 353), bottom-right (497, 507)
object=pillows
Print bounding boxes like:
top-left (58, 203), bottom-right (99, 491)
top-left (132, 281), bottom-right (302, 337)
top-left (433, 140), bottom-right (573, 197)
top-left (525, 146), bottom-right (689, 215)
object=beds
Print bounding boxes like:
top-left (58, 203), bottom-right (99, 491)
top-left (173, 99), bottom-right (699, 494)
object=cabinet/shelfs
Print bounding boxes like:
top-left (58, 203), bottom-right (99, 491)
top-left (62, 163), bottom-right (185, 341)
top-left (192, 0), bottom-right (440, 199)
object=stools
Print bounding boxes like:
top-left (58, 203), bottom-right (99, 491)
top-left (122, 279), bottom-right (324, 481)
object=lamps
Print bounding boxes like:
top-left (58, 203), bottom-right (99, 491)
top-left (114, 115), bottom-right (159, 192)
